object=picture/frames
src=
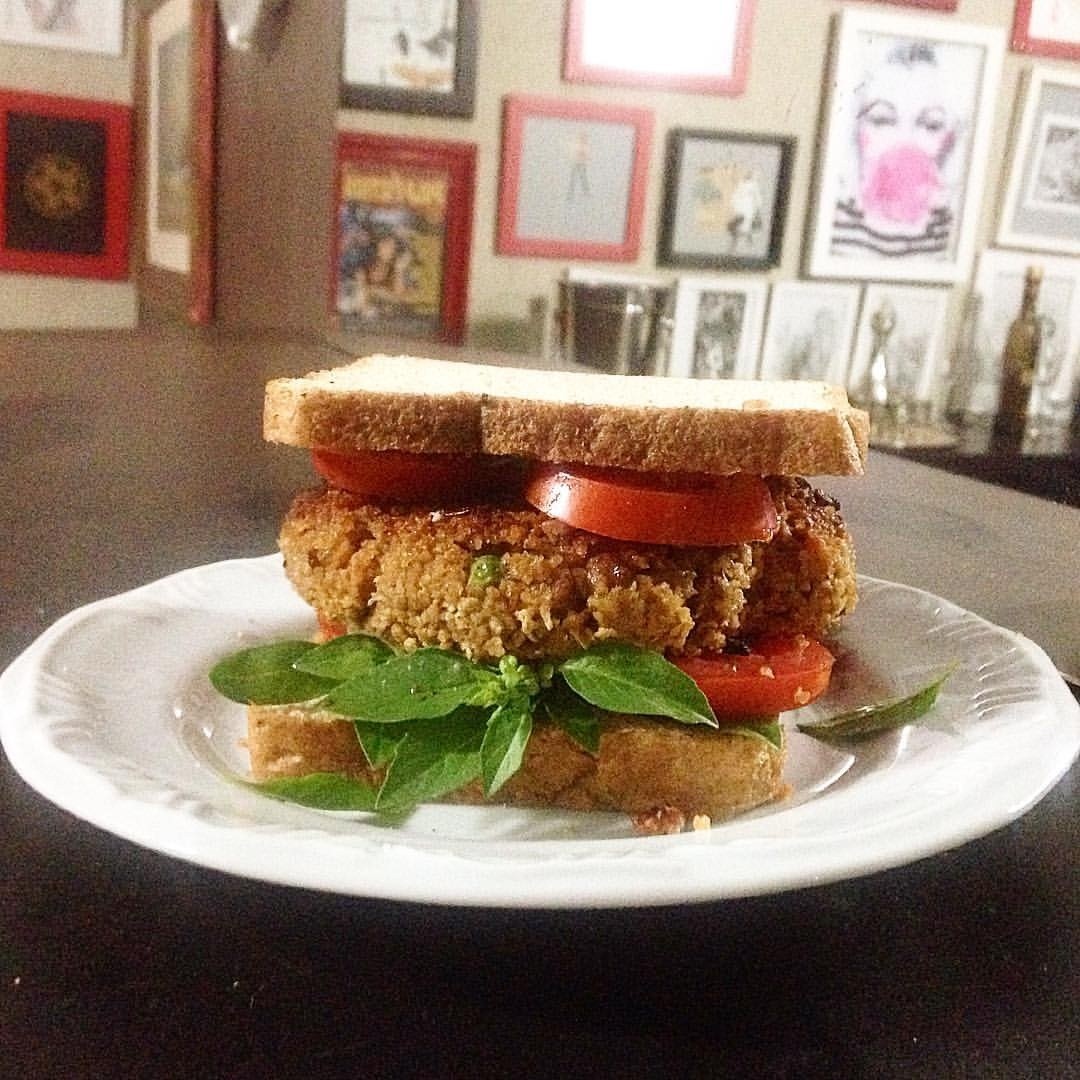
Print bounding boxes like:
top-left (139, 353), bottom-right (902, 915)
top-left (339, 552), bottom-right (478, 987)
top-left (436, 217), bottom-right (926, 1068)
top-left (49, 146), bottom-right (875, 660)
top-left (667, 255), bottom-right (1080, 420)
top-left (563, 0), bottom-right (772, 101)
top-left (1010, 0), bottom-right (1079, 62)
top-left (799, 11), bottom-right (1004, 286)
top-left (136, 0), bottom-right (220, 327)
top-left (339, 1), bottom-right (480, 118)
top-left (495, 94), bottom-right (657, 261)
top-left (330, 132), bottom-right (478, 347)
top-left (667, 245), bottom-right (1079, 422)
top-left (657, 128), bottom-right (797, 275)
top-left (0, 0), bottom-right (129, 57)
top-left (1, 89), bottom-right (135, 284)
top-left (993, 66), bottom-right (1079, 255)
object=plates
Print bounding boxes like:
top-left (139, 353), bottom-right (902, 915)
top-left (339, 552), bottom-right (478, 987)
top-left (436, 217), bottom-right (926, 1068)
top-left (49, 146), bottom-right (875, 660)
top-left (0, 551), bottom-right (1078, 910)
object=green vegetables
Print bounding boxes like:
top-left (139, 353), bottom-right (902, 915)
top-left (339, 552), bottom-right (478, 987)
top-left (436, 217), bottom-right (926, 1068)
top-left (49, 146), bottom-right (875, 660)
top-left (796, 663), bottom-right (962, 742)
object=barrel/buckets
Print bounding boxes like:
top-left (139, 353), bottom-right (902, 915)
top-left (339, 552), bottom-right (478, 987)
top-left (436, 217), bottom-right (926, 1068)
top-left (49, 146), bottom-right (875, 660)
top-left (555, 278), bottom-right (672, 374)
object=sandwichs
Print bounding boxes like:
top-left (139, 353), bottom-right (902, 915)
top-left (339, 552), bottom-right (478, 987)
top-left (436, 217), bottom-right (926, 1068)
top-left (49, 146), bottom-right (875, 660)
top-left (208, 353), bottom-right (871, 823)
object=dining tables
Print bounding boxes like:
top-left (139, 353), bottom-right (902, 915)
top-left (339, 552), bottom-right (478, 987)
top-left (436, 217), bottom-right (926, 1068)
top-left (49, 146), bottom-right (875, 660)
top-left (0, 325), bottom-right (1079, 1080)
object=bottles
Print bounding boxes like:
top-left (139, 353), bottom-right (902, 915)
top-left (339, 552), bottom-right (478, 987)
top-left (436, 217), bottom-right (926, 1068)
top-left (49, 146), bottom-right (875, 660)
top-left (941, 294), bottom-right (983, 424)
top-left (860, 312), bottom-right (898, 441)
top-left (991, 271), bottom-right (1044, 452)
